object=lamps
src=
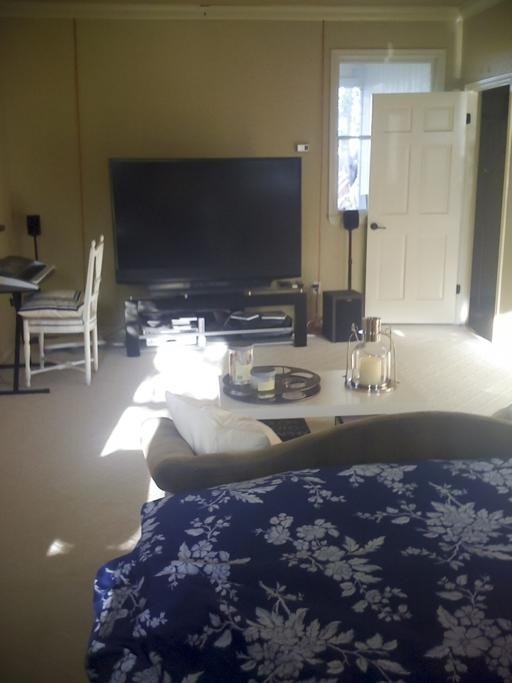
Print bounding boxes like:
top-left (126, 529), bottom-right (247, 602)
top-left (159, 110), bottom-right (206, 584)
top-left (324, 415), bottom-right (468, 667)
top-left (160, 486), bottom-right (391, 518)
top-left (340, 210), bottom-right (358, 287)
top-left (26, 215), bottom-right (45, 262)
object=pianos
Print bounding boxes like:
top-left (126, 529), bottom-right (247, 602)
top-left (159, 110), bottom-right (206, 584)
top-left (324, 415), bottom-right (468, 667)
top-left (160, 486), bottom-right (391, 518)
top-left (0, 255), bottom-right (56, 292)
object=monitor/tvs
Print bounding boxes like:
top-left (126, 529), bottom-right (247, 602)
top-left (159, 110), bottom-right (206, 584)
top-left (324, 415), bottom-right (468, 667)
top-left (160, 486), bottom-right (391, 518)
top-left (108, 156), bottom-right (303, 291)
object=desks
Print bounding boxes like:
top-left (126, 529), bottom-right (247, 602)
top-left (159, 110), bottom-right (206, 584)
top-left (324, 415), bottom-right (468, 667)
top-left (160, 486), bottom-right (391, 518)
top-left (168, 371), bottom-right (430, 419)
top-left (122, 283), bottom-right (308, 356)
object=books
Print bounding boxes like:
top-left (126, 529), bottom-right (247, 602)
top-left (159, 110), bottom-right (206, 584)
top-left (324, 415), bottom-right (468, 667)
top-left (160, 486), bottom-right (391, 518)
top-left (261, 310), bottom-right (289, 320)
top-left (229, 309), bottom-right (260, 322)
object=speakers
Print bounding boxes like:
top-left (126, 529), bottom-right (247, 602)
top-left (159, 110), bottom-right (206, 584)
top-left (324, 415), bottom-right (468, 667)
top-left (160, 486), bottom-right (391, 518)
top-left (27, 215), bottom-right (42, 236)
top-left (321, 290), bottom-right (363, 341)
top-left (343, 210), bottom-right (359, 230)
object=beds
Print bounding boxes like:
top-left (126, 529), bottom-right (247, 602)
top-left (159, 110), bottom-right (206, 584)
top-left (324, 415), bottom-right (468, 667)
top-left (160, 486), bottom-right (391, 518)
top-left (87, 459), bottom-right (511, 682)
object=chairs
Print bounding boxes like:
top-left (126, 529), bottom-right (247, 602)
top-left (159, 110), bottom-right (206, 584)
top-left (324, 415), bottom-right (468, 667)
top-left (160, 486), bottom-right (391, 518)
top-left (18, 232), bottom-right (105, 387)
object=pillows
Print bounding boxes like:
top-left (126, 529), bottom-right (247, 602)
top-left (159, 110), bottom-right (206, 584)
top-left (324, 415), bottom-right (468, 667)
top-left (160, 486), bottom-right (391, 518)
top-left (169, 405), bottom-right (282, 452)
top-left (18, 289), bottom-right (82, 320)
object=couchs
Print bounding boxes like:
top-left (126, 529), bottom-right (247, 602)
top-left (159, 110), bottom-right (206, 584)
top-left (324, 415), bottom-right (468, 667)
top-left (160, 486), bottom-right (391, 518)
top-left (139, 407), bottom-right (512, 492)
